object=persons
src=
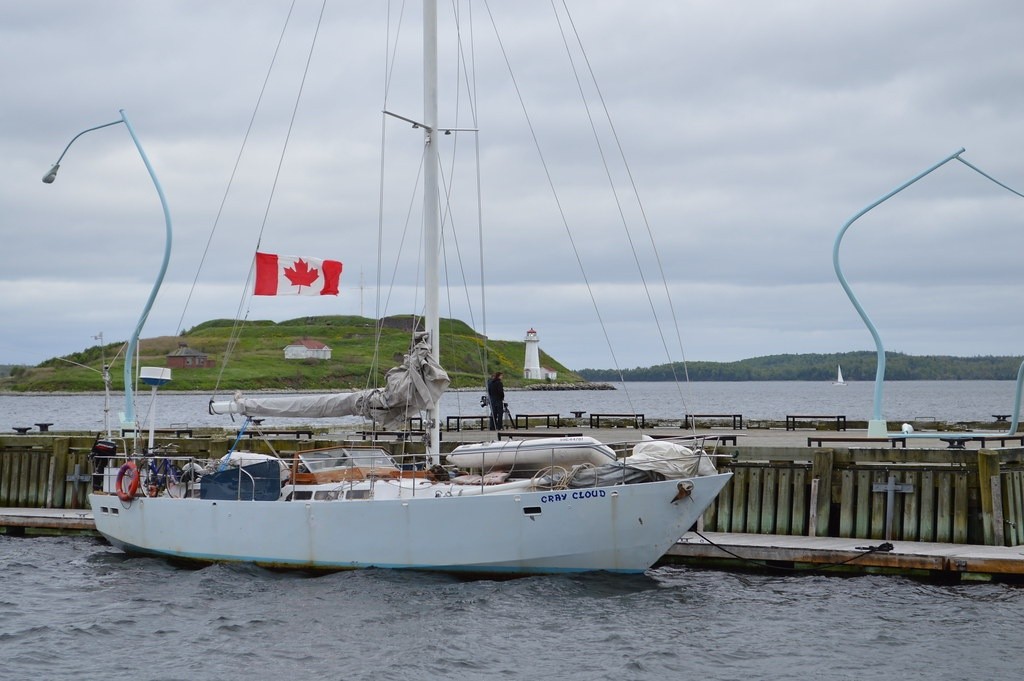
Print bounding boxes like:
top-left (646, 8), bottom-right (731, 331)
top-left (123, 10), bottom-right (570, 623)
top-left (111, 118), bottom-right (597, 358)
top-left (486, 372), bottom-right (504, 431)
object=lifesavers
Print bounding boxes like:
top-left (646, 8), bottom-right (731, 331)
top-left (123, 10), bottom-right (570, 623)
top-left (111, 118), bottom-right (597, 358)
top-left (116, 462), bottom-right (140, 500)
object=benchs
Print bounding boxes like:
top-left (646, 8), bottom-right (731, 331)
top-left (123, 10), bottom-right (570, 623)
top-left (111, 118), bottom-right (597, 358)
top-left (685, 414), bottom-right (744, 431)
top-left (963, 436), bottom-right (1024, 449)
top-left (121, 429), bottom-right (193, 438)
top-left (373, 417), bottom-right (423, 431)
top-left (356, 431), bottom-right (426, 440)
top-left (786, 415), bottom-right (846, 432)
top-left (497, 433), bottom-right (583, 442)
top-left (446, 416), bottom-right (495, 431)
top-left (515, 414), bottom-right (559, 429)
top-left (590, 413), bottom-right (645, 430)
top-left (650, 435), bottom-right (737, 446)
top-left (237, 430), bottom-right (315, 453)
top-left (806, 436), bottom-right (907, 464)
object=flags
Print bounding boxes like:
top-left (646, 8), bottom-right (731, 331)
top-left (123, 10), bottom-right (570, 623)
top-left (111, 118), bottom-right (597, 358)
top-left (254, 251), bottom-right (343, 296)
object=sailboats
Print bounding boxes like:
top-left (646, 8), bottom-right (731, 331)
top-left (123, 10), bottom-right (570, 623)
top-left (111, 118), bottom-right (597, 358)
top-left (830, 364), bottom-right (847, 386)
top-left (86, 2), bottom-right (735, 578)
